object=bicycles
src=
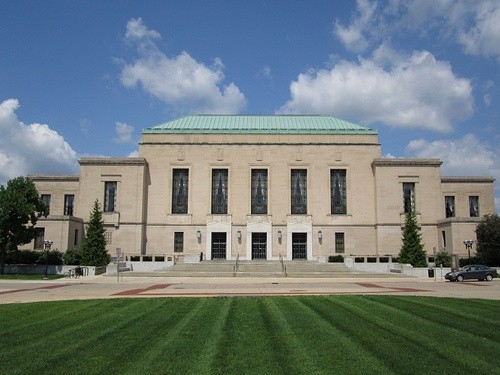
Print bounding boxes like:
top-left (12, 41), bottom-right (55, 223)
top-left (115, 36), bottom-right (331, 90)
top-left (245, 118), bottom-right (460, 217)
top-left (63, 268), bottom-right (79, 279)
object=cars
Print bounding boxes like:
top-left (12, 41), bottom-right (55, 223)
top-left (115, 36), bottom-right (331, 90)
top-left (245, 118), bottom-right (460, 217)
top-left (445, 264), bottom-right (498, 282)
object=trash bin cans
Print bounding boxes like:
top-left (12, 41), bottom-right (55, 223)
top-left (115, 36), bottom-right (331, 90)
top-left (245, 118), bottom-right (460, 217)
top-left (75, 268), bottom-right (81, 276)
top-left (428, 269), bottom-right (434, 277)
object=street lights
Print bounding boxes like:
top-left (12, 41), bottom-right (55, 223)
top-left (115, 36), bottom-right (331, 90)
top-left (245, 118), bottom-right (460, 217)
top-left (463, 240), bottom-right (474, 265)
top-left (42, 239), bottom-right (53, 279)
